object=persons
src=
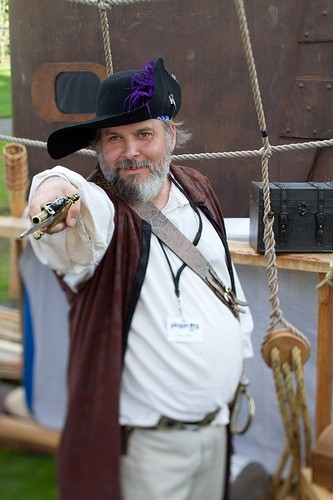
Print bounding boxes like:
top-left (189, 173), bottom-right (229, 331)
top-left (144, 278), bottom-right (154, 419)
top-left (24, 55), bottom-right (255, 500)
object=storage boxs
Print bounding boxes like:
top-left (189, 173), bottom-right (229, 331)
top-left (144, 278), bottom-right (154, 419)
top-left (248, 181), bottom-right (333, 255)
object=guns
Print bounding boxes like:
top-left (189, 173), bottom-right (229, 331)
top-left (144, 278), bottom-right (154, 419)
top-left (20, 192), bottom-right (80, 240)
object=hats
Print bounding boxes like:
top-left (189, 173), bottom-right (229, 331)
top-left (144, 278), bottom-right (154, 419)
top-left (46, 57), bottom-right (181, 161)
